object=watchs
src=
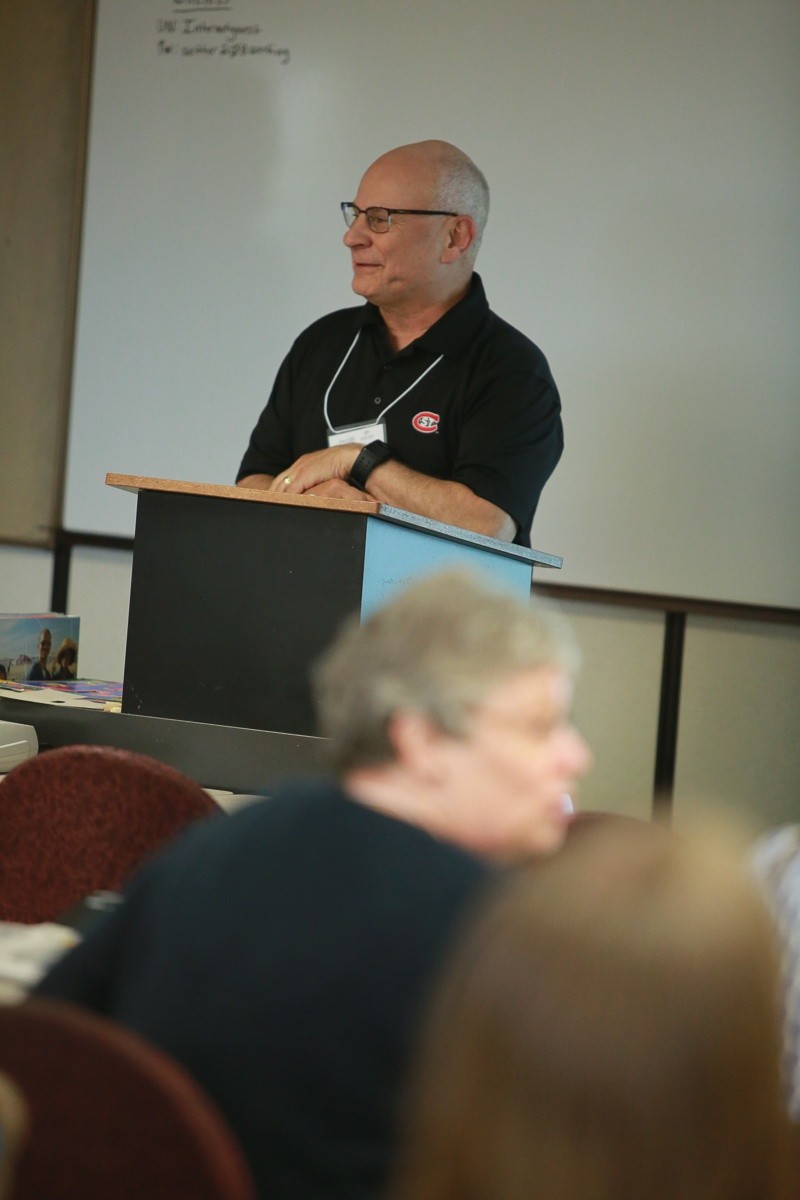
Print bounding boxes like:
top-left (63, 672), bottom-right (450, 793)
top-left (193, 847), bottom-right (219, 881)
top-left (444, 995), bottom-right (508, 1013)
top-left (348, 440), bottom-right (391, 491)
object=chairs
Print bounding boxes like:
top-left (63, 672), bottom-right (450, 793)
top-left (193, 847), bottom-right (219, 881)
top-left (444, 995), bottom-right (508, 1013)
top-left (0, 747), bottom-right (261, 1200)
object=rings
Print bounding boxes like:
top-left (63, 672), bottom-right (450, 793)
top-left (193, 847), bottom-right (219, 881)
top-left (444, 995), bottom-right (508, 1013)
top-left (285, 478), bottom-right (290, 484)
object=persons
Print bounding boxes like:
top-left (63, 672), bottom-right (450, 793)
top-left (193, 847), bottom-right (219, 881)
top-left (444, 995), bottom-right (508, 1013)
top-left (0, 628), bottom-right (78, 681)
top-left (21, 571), bottom-right (800, 1200)
top-left (235, 140), bottom-right (564, 549)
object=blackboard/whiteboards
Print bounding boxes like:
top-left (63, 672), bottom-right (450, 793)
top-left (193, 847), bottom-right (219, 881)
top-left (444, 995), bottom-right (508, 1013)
top-left (58, 1), bottom-right (799, 610)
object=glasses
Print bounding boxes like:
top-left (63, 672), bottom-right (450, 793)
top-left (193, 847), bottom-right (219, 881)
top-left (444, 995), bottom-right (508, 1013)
top-left (341, 202), bottom-right (459, 233)
